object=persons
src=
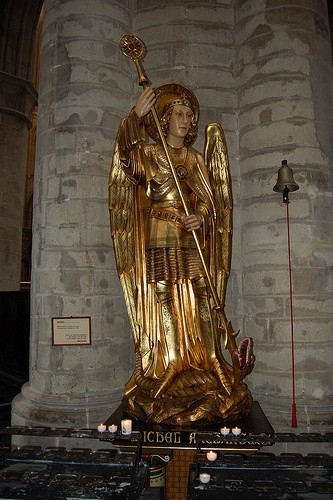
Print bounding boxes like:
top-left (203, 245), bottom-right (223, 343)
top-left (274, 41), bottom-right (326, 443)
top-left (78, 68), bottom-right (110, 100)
top-left (108, 84), bottom-right (253, 421)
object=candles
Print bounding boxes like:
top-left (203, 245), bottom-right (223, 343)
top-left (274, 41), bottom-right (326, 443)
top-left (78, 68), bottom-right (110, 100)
top-left (231, 427), bottom-right (241, 436)
top-left (200, 474), bottom-right (210, 483)
top-left (108, 424), bottom-right (117, 433)
top-left (220, 427), bottom-right (230, 435)
top-left (121, 419), bottom-right (132, 435)
top-left (98, 423), bottom-right (106, 433)
top-left (207, 451), bottom-right (217, 460)
